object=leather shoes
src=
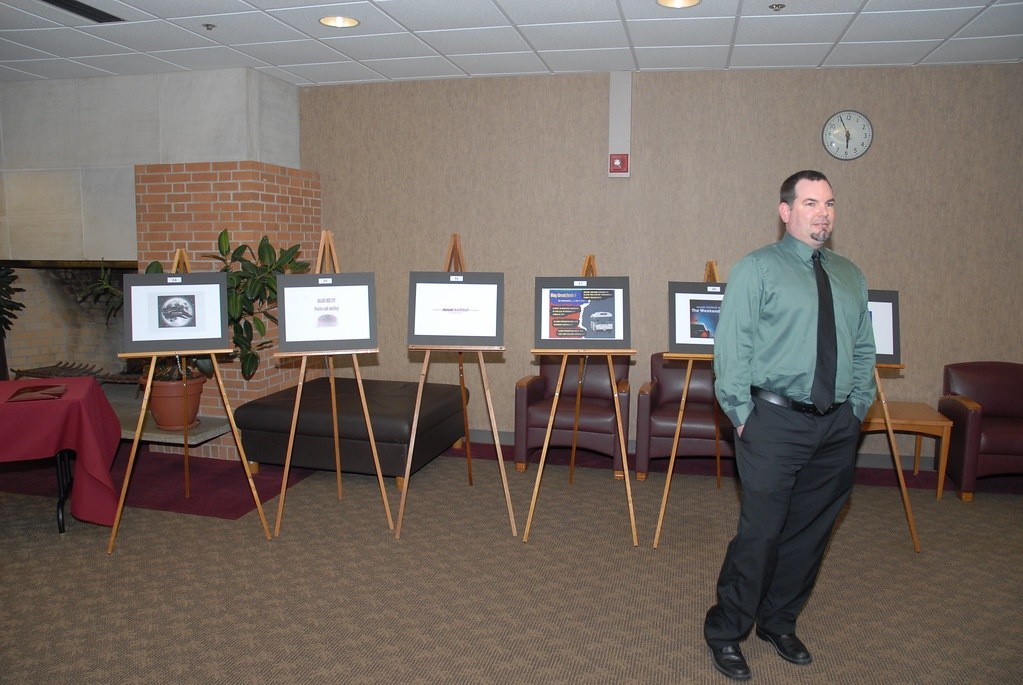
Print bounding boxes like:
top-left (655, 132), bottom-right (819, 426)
top-left (756, 624), bottom-right (813, 665)
top-left (711, 643), bottom-right (752, 680)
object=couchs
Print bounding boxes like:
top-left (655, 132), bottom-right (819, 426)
top-left (513, 338), bottom-right (633, 479)
top-left (232, 375), bottom-right (472, 491)
top-left (933, 360), bottom-right (1023, 503)
top-left (634, 345), bottom-right (737, 483)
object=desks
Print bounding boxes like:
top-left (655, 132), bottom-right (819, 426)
top-left (0, 377), bottom-right (126, 527)
top-left (860, 397), bottom-right (954, 499)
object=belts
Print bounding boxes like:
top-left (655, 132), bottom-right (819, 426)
top-left (749, 386), bottom-right (850, 418)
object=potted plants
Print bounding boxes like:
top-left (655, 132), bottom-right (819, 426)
top-left (123, 232), bottom-right (310, 433)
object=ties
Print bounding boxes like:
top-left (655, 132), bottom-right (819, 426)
top-left (809, 250), bottom-right (837, 414)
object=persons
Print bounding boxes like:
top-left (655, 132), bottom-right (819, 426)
top-left (702, 168), bottom-right (877, 681)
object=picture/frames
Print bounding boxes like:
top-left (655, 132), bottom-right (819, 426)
top-left (275, 272), bottom-right (380, 351)
top-left (122, 272), bottom-right (231, 353)
top-left (668, 280), bottom-right (729, 356)
top-left (865, 287), bottom-right (903, 366)
top-left (407, 270), bottom-right (507, 349)
top-left (533, 275), bottom-right (634, 350)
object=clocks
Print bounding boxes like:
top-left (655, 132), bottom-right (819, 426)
top-left (822, 110), bottom-right (875, 160)
